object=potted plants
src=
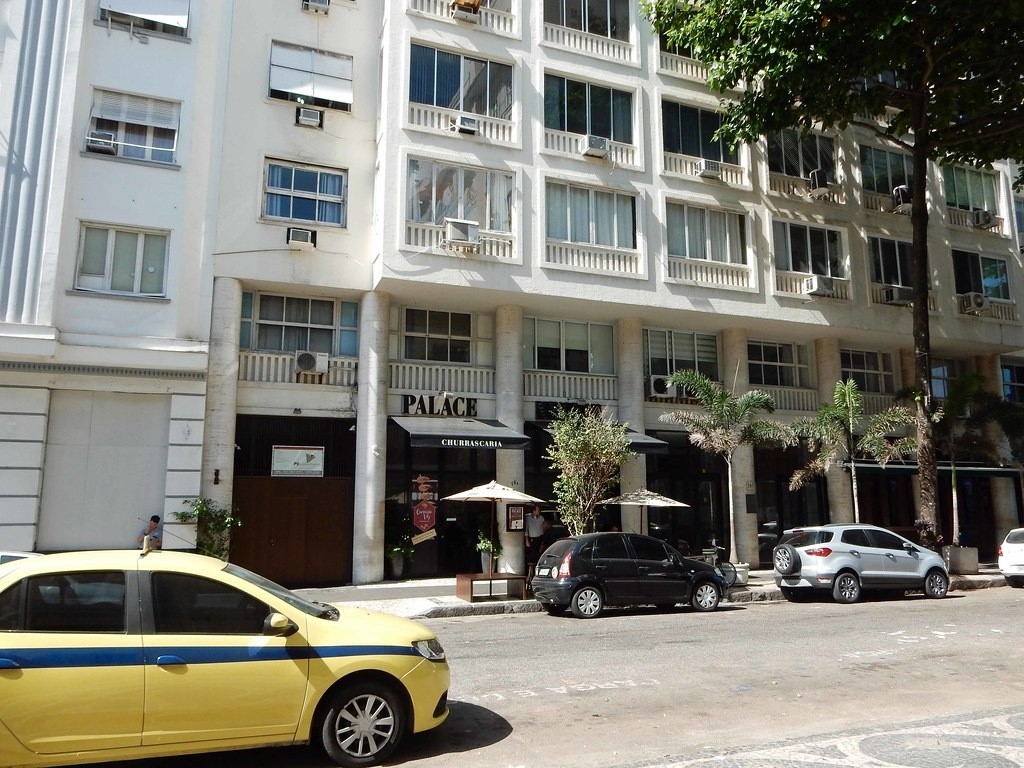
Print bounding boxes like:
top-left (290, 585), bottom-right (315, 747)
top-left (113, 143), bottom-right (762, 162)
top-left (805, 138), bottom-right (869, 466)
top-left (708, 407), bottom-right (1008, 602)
top-left (477, 529), bottom-right (502, 576)
top-left (660, 370), bottom-right (799, 584)
top-left (886, 364), bottom-right (1024, 572)
top-left (388, 540), bottom-right (415, 575)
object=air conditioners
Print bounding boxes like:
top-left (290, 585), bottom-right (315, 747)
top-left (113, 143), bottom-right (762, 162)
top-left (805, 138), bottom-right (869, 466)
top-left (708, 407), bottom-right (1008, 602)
top-left (87, 131), bottom-right (116, 154)
top-left (581, 134), bottom-right (610, 157)
top-left (685, 379), bottom-right (702, 399)
top-left (974, 210), bottom-right (996, 228)
top-left (446, 217), bottom-right (479, 242)
top-left (696, 159), bottom-right (721, 177)
top-left (650, 374), bottom-right (677, 397)
top-left (453, 5), bottom-right (474, 14)
top-left (457, 116), bottom-right (478, 131)
top-left (804, 276), bottom-right (833, 294)
top-left (294, 349), bottom-right (328, 373)
top-left (299, 108), bottom-right (319, 127)
top-left (963, 292), bottom-right (989, 314)
top-left (102, 9), bottom-right (144, 28)
top-left (289, 229), bottom-right (312, 243)
top-left (883, 287), bottom-right (914, 304)
top-left (308, 0), bottom-right (330, 12)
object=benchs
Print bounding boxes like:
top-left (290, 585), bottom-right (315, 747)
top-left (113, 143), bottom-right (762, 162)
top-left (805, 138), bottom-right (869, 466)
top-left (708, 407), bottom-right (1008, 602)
top-left (456, 572), bottom-right (526, 603)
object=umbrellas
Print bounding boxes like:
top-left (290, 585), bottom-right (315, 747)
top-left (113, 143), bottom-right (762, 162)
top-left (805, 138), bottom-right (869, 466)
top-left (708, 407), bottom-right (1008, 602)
top-left (595, 486), bottom-right (691, 533)
top-left (440, 480), bottom-right (545, 598)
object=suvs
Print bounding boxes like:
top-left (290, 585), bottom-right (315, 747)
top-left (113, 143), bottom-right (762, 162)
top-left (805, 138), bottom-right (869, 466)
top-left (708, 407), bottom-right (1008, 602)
top-left (772, 521), bottom-right (951, 604)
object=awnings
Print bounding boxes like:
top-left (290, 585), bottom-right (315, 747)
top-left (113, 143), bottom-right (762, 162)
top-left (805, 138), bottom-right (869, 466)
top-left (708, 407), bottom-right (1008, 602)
top-left (844, 434), bottom-right (1020, 471)
top-left (388, 416), bottom-right (532, 450)
top-left (524, 422), bottom-right (669, 454)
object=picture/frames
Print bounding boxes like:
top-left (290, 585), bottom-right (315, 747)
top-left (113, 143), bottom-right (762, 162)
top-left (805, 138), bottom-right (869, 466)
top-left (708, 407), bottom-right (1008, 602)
top-left (506, 504), bottom-right (527, 533)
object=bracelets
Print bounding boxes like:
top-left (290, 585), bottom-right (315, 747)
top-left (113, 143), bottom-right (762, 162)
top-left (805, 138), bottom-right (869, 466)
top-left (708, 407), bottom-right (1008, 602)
top-left (144, 533), bottom-right (148, 536)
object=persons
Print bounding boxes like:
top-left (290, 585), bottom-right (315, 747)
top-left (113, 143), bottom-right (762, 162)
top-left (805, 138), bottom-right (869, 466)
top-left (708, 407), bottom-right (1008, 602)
top-left (137, 515), bottom-right (162, 550)
top-left (920, 524), bottom-right (944, 556)
top-left (525, 506), bottom-right (551, 562)
top-left (609, 521), bottom-right (618, 530)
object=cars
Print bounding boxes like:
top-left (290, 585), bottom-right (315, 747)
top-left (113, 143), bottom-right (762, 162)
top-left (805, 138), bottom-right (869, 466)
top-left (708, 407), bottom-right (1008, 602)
top-left (0, 548), bottom-right (453, 768)
top-left (997, 527), bottom-right (1024, 589)
top-left (529, 530), bottom-right (728, 618)
